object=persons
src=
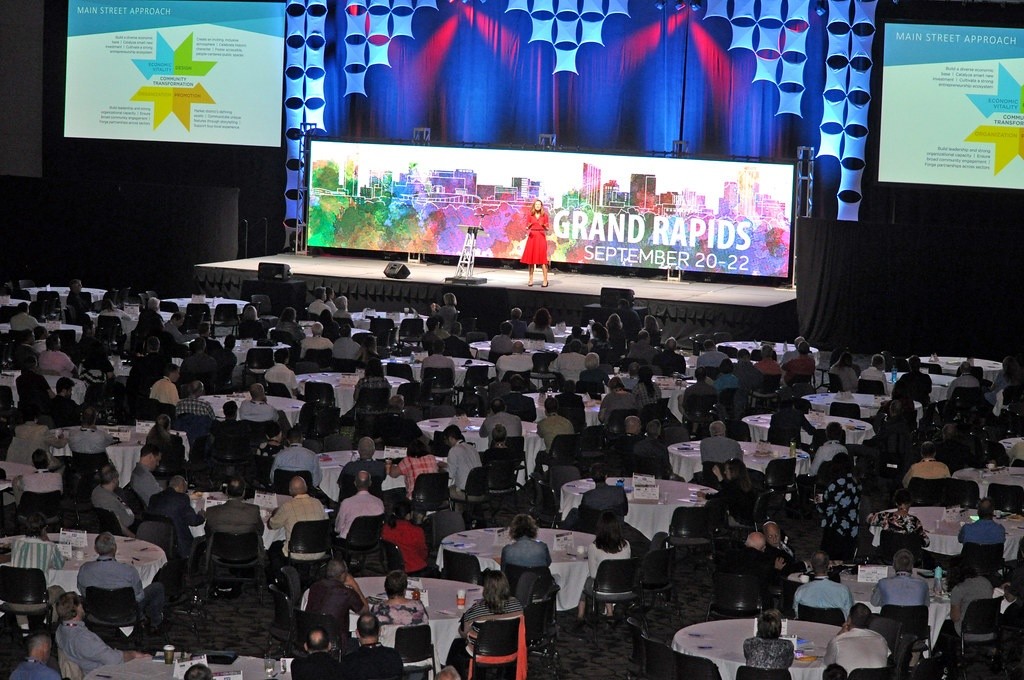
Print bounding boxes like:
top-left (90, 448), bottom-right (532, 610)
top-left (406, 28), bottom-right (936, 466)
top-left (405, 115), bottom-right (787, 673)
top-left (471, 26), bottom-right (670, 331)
top-left (263, 280), bottom-right (656, 679)
top-left (637, 312), bottom-right (1024, 680)
top-left (520, 198), bottom-right (549, 288)
top-left (0, 276), bottom-right (285, 680)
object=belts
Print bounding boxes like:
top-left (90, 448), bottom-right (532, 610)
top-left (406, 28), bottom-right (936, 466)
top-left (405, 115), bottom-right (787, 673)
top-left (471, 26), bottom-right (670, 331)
top-left (461, 489), bottom-right (466, 493)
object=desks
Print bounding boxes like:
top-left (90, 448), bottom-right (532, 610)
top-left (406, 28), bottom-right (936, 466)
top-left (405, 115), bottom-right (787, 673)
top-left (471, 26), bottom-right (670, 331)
top-left (434, 526), bottom-right (597, 611)
top-left (741, 414), bottom-right (881, 445)
top-left (905, 357), bottom-right (1003, 388)
top-left (603, 375), bottom-right (697, 423)
top-left (469, 339), bottom-right (564, 361)
top-left (267, 326), bottom-right (373, 340)
top-left (869, 507), bottom-right (1024, 577)
top-left (186, 492), bottom-right (292, 551)
top-left (0, 533), bottom-right (168, 636)
top-left (294, 373), bottom-right (410, 418)
top-left (951, 467), bottom-right (1024, 500)
top-left (999, 437), bottom-right (1024, 449)
top-left (0, 298), bottom-right (32, 306)
top-left (183, 338), bottom-right (292, 365)
top-left (0, 371), bottom-right (86, 409)
top-left (0, 323), bottom-right (83, 344)
top-left (715, 341), bottom-right (821, 368)
top-left (550, 326), bottom-right (590, 344)
top-left (109, 358), bottom-right (183, 377)
top-left (521, 393), bottom-right (602, 427)
top-left (160, 298), bottom-right (250, 325)
top-left (801, 393), bottom-right (923, 428)
top-left (81, 655), bottom-right (292, 680)
top-left (316, 450), bottom-right (456, 503)
top-left (350, 312), bottom-right (430, 342)
top-left (21, 287), bottom-right (108, 311)
top-left (198, 393), bottom-right (306, 429)
top-left (559, 476), bottom-right (730, 543)
top-left (417, 417), bottom-right (549, 491)
top-left (859, 372), bottom-right (959, 425)
top-left (788, 564), bottom-right (952, 659)
top-left (683, 357), bottom-right (758, 380)
top-left (86, 311), bottom-right (174, 338)
top-left (667, 441), bottom-right (811, 485)
top-left (49, 425), bottom-right (191, 490)
top-left (299, 576), bottom-right (485, 666)
top-left (0, 460), bottom-right (38, 506)
top-left (379, 357), bottom-right (497, 405)
top-left (671, 619), bottom-right (893, 680)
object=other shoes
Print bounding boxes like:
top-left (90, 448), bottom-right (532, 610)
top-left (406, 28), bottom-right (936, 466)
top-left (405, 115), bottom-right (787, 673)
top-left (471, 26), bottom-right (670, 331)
top-left (604, 615), bottom-right (616, 623)
top-left (573, 618), bottom-right (587, 632)
top-left (529, 284), bottom-right (533, 287)
top-left (542, 282), bottom-right (548, 287)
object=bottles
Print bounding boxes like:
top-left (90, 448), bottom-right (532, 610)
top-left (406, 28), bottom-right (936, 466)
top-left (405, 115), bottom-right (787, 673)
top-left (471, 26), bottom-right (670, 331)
top-left (223, 483), bottom-right (227, 493)
top-left (891, 365), bottom-right (897, 381)
top-left (412, 588), bottom-right (420, 600)
top-left (390, 356), bottom-right (396, 363)
top-left (616, 477), bottom-right (625, 487)
top-left (934, 566), bottom-right (943, 595)
top-left (755, 441), bottom-right (771, 455)
top-left (809, 409), bottom-right (825, 423)
top-left (946, 505), bottom-right (960, 522)
top-left (385, 460), bottom-right (399, 475)
top-left (538, 387), bottom-right (553, 406)
top-left (790, 438), bottom-right (796, 458)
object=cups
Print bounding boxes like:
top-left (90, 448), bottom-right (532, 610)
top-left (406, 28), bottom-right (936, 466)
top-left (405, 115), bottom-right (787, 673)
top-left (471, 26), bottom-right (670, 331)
top-left (986, 463), bottom-right (994, 469)
top-left (935, 519), bottom-right (939, 529)
top-left (163, 645), bottom-right (175, 664)
top-left (73, 551), bottom-right (83, 560)
top-left (811, 416), bottom-right (822, 426)
top-left (457, 590), bottom-right (466, 610)
top-left (578, 545), bottom-right (584, 553)
top-left (404, 307), bottom-right (409, 316)
top-left (996, 509), bottom-right (1001, 518)
top-left (265, 659), bottom-right (275, 679)
top-left (497, 529), bottom-right (505, 537)
top-left (663, 492), bottom-right (667, 504)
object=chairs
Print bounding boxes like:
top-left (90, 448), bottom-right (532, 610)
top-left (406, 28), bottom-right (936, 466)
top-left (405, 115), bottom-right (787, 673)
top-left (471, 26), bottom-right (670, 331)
top-left (0, 278), bottom-right (1024, 680)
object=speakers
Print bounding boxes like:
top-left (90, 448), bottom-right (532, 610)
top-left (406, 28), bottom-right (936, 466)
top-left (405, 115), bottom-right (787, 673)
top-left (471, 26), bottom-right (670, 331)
top-left (258, 263), bottom-right (292, 281)
top-left (384, 262), bottom-right (410, 278)
top-left (600, 288), bottom-right (635, 307)
